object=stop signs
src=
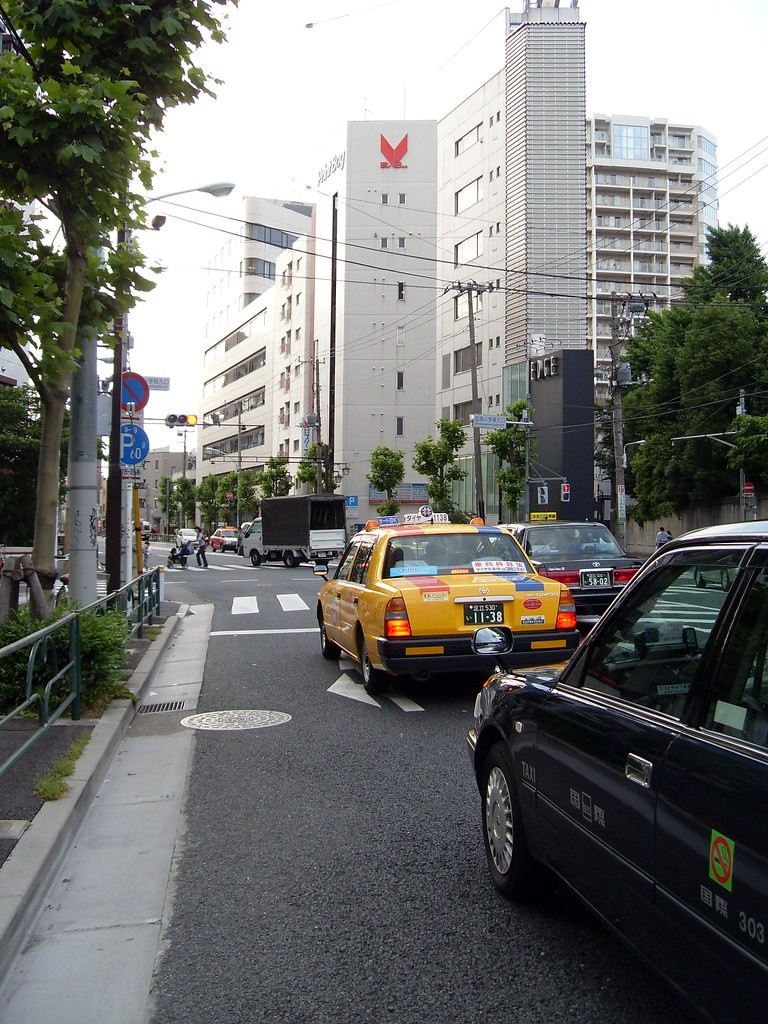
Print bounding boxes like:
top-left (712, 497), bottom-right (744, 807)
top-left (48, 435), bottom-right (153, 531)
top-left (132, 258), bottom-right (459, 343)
top-left (744, 482), bottom-right (755, 494)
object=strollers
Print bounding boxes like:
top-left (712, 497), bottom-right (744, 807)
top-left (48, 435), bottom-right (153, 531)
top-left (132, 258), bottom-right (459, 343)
top-left (166, 540), bottom-right (196, 570)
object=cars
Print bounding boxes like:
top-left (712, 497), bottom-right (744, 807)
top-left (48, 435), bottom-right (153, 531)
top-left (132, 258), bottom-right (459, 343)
top-left (175, 528), bottom-right (200, 549)
top-left (57, 534), bottom-right (65, 555)
top-left (464, 521), bottom-right (766, 1024)
top-left (208, 528), bottom-right (241, 553)
top-left (315, 506), bottom-right (579, 696)
top-left (494, 511), bottom-right (643, 618)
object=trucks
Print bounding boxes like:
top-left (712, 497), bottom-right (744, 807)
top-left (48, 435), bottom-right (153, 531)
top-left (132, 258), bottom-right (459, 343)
top-left (239, 494), bottom-right (350, 568)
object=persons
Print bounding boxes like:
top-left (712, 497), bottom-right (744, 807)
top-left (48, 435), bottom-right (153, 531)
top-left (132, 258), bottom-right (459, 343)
top-left (167, 548), bottom-right (176, 568)
top-left (188, 526), bottom-right (208, 568)
top-left (132, 528), bottom-right (151, 580)
top-left (655, 527), bottom-right (672, 549)
top-left (151, 528), bottom-right (155, 541)
top-left (203, 530), bottom-right (210, 545)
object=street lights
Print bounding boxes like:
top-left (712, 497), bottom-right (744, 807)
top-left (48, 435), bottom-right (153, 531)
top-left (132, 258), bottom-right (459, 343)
top-left (328, 459), bottom-right (351, 492)
top-left (98, 180), bottom-right (237, 227)
top-left (613, 441), bottom-right (646, 550)
top-left (206, 445), bottom-right (237, 476)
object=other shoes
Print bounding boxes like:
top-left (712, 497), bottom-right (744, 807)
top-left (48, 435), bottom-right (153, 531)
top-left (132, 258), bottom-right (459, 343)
top-left (203, 565), bottom-right (208, 568)
top-left (196, 564), bottom-right (201, 567)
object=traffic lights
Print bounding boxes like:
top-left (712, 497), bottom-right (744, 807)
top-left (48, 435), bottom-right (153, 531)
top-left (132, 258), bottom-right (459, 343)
top-left (561, 484), bottom-right (570, 500)
top-left (540, 488), bottom-right (546, 502)
top-left (165, 413), bottom-right (199, 428)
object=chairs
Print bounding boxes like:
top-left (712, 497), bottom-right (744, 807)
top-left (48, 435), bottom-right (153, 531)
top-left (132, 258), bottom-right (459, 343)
top-left (391, 547), bottom-right (415, 563)
top-left (425, 541), bottom-right (449, 566)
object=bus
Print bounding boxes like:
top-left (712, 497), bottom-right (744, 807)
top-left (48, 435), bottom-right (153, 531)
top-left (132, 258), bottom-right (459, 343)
top-left (139, 522), bottom-right (152, 540)
